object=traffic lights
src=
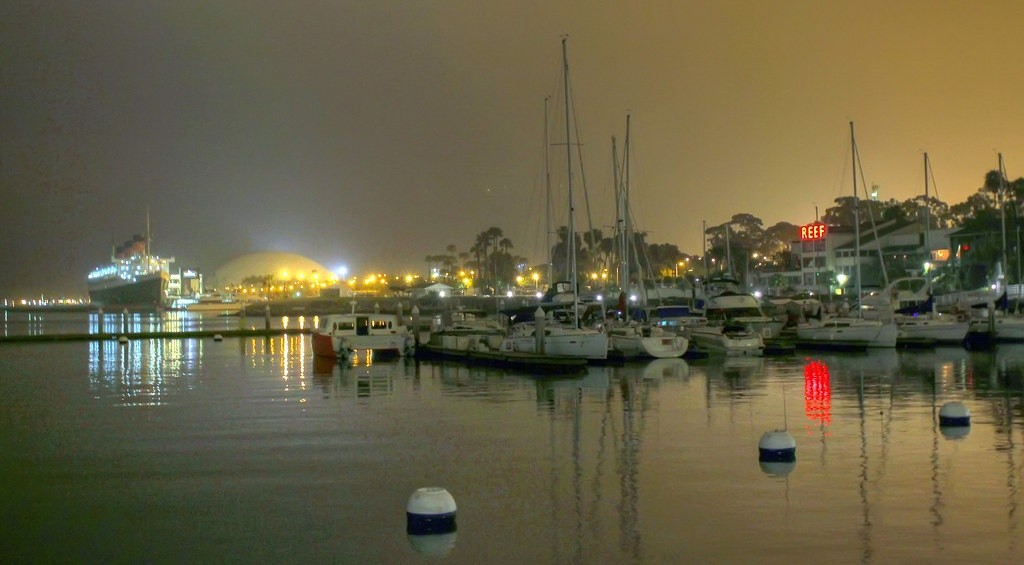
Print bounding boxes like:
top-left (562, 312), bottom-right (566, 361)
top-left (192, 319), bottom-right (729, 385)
top-left (815, 272), bottom-right (820, 277)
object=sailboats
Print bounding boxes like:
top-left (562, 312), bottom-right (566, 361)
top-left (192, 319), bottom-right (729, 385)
top-left (964, 154), bottom-right (1024, 346)
top-left (510, 38), bottom-right (608, 362)
top-left (611, 136), bottom-right (707, 326)
top-left (797, 121), bottom-right (896, 347)
top-left (582, 114), bottom-right (689, 358)
top-left (880, 153), bottom-right (970, 343)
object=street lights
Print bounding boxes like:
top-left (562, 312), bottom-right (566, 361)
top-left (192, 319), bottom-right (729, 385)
top-left (616, 264), bottom-right (619, 285)
top-left (534, 274), bottom-right (537, 288)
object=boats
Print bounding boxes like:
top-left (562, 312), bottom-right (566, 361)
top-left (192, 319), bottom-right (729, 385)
top-left (688, 277), bottom-right (787, 344)
top-left (311, 313), bottom-right (416, 359)
top-left (87, 204), bottom-right (170, 307)
top-left (692, 324), bottom-right (766, 357)
top-left (186, 296), bottom-right (241, 315)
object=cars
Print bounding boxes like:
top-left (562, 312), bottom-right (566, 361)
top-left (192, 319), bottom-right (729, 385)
top-left (799, 290), bottom-right (816, 298)
